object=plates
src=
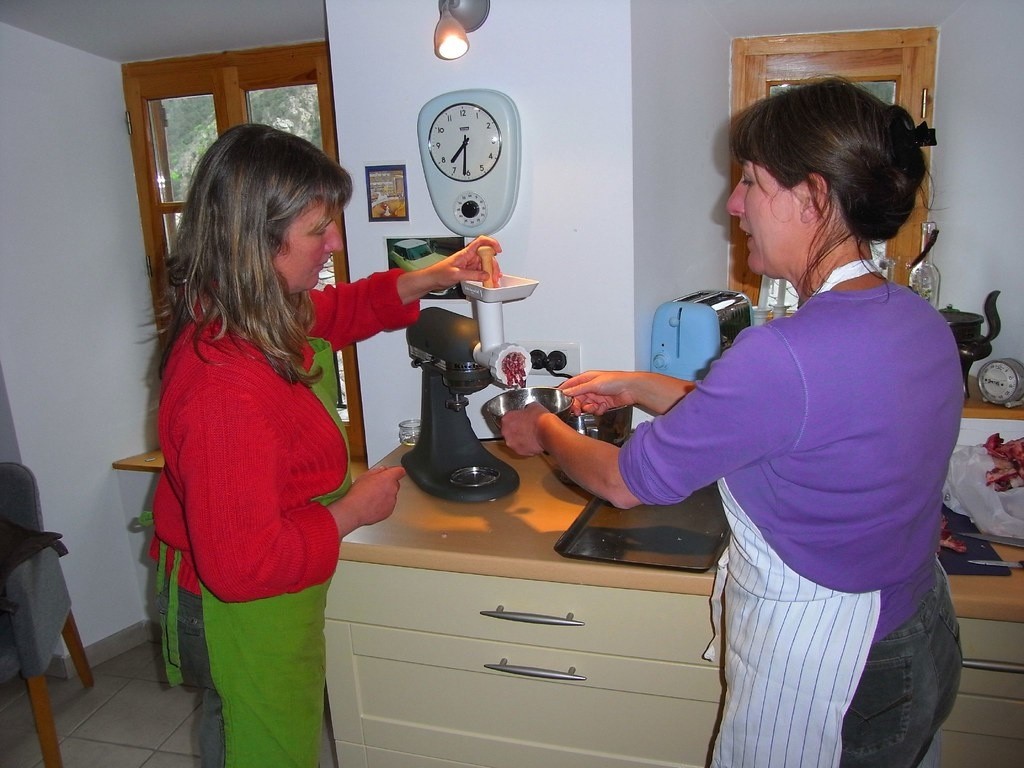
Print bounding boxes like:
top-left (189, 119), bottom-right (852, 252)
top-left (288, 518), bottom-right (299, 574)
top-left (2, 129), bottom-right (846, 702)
top-left (450, 466), bottom-right (500, 489)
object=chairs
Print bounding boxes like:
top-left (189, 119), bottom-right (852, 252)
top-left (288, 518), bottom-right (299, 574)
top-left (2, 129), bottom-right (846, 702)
top-left (0, 461), bottom-right (92, 767)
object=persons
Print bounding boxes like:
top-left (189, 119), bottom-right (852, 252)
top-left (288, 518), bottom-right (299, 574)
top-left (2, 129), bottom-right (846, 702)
top-left (137, 123), bottom-right (499, 768)
top-left (502, 81), bottom-right (964, 768)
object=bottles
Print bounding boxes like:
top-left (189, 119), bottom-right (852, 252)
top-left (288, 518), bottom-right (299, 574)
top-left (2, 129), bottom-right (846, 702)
top-left (909, 221), bottom-right (940, 310)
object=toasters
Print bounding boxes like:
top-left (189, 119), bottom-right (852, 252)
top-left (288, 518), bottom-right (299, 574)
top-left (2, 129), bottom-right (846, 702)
top-left (651, 290), bottom-right (753, 383)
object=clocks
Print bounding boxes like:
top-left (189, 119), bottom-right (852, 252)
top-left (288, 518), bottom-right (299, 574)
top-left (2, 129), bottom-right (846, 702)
top-left (417, 88), bottom-right (521, 236)
top-left (974, 359), bottom-right (1024, 409)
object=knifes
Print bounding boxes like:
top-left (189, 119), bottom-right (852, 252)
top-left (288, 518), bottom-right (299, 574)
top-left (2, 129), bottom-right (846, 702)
top-left (958, 532), bottom-right (1024, 548)
top-left (968, 560), bottom-right (1024, 568)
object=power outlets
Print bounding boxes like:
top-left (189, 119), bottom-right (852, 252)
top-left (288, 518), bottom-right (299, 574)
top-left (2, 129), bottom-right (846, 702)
top-left (515, 340), bottom-right (580, 378)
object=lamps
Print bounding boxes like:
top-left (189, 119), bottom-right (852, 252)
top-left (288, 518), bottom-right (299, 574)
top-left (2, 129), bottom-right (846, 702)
top-left (431, 1), bottom-right (490, 61)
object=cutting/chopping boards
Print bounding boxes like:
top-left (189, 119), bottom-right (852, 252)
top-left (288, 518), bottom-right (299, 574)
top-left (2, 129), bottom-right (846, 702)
top-left (937, 502), bottom-right (1012, 576)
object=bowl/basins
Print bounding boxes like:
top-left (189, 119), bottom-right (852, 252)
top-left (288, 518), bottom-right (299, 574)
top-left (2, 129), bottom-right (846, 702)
top-left (567, 405), bottom-right (633, 447)
top-left (487, 387), bottom-right (574, 430)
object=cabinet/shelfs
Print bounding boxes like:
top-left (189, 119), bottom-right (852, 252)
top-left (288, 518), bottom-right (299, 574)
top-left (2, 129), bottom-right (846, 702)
top-left (323, 553), bottom-right (1023, 768)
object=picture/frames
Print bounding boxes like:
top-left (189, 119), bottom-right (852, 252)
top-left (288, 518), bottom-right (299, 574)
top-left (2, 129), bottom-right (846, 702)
top-left (365, 164), bottom-right (408, 223)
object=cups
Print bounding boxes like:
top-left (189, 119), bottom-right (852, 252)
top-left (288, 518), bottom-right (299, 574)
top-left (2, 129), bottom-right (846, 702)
top-left (398, 419), bottom-right (421, 446)
